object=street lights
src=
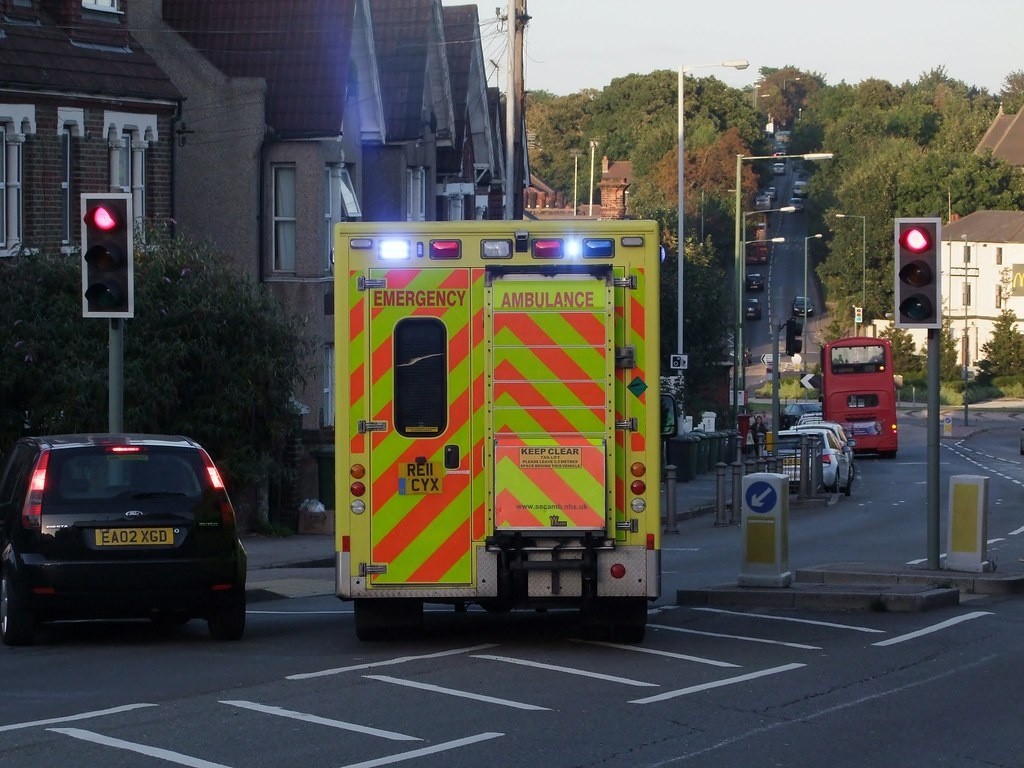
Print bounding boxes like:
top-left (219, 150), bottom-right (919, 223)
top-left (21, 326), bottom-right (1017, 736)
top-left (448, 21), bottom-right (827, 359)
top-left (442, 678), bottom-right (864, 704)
top-left (803, 233), bottom-right (823, 355)
top-left (677, 59), bottom-right (750, 414)
top-left (836, 213), bottom-right (865, 306)
top-left (738, 205), bottom-right (798, 390)
top-left (733, 151), bottom-right (835, 434)
top-left (739, 237), bottom-right (786, 385)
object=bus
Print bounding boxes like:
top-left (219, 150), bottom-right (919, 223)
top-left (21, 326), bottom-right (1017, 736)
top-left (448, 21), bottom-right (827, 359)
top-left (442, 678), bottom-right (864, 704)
top-left (820, 336), bottom-right (899, 460)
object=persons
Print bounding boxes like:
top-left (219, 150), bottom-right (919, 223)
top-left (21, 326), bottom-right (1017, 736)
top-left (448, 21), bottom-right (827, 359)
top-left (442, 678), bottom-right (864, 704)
top-left (748, 415), bottom-right (769, 459)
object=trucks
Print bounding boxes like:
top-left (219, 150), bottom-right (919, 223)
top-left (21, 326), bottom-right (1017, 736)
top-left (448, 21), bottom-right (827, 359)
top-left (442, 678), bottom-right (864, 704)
top-left (334, 221), bottom-right (660, 645)
top-left (746, 212), bottom-right (772, 264)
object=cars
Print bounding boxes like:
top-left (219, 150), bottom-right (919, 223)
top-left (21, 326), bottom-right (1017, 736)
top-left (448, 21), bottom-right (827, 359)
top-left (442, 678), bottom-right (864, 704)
top-left (789, 422), bottom-right (855, 481)
top-left (763, 186), bottom-right (778, 201)
top-left (773, 152), bottom-right (787, 161)
top-left (744, 298), bottom-right (762, 320)
top-left (0, 431), bottom-right (247, 644)
top-left (781, 402), bottom-right (823, 425)
top-left (789, 198), bottom-right (804, 213)
top-left (762, 428), bottom-right (852, 496)
top-left (746, 273), bottom-right (766, 292)
top-left (791, 295), bottom-right (815, 317)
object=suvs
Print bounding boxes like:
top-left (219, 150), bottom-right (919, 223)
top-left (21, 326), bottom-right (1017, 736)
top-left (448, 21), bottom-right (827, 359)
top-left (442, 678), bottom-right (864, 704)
top-left (772, 162), bottom-right (785, 175)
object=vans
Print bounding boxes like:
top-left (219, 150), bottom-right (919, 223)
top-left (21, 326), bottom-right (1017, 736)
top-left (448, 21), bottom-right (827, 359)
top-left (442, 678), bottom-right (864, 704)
top-left (792, 181), bottom-right (809, 197)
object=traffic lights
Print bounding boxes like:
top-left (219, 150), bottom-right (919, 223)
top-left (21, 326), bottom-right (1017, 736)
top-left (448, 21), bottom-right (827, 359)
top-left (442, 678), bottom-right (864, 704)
top-left (79, 192), bottom-right (136, 319)
top-left (856, 307), bottom-right (862, 324)
top-left (894, 216), bottom-right (943, 331)
